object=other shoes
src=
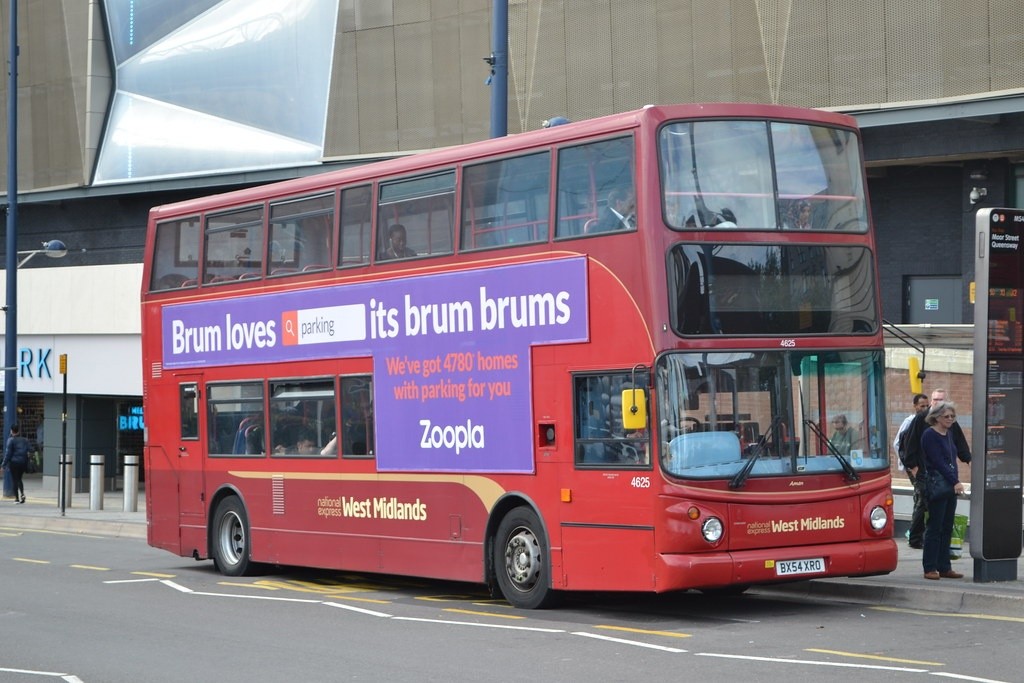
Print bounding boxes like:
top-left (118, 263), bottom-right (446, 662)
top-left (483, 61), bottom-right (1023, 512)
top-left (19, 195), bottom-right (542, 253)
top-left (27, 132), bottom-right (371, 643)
top-left (924, 569), bottom-right (963, 580)
top-left (13, 494), bottom-right (26, 505)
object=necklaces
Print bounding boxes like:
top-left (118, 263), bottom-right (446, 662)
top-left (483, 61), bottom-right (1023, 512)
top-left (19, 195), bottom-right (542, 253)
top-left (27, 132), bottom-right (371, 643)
top-left (392, 248), bottom-right (405, 258)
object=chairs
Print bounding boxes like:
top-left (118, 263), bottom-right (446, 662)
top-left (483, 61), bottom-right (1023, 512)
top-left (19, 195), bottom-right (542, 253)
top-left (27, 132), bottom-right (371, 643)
top-left (181, 279), bottom-right (198, 287)
top-left (281, 424), bottom-right (314, 447)
top-left (210, 277), bottom-right (235, 283)
top-left (303, 265), bottom-right (329, 272)
top-left (232, 417), bottom-right (259, 454)
top-left (271, 268), bottom-right (298, 275)
top-left (238, 273), bottom-right (260, 280)
top-left (159, 273), bottom-right (190, 290)
top-left (244, 424), bottom-right (264, 454)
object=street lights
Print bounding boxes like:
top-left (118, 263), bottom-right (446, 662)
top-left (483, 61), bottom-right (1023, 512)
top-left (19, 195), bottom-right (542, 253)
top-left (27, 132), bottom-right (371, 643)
top-left (3, 239), bottom-right (69, 502)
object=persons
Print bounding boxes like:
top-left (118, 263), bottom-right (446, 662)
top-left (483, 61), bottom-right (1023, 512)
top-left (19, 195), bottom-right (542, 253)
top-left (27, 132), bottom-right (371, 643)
top-left (589, 189), bottom-right (637, 233)
top-left (36, 414), bottom-right (43, 453)
top-left (827, 415), bottom-right (859, 455)
top-left (275, 377), bottom-right (374, 454)
top-left (787, 199), bottom-right (813, 230)
top-left (893, 388), bottom-right (971, 580)
top-left (2, 424), bottom-right (31, 503)
top-left (377, 224), bottom-right (418, 260)
top-left (871, 425), bottom-right (880, 458)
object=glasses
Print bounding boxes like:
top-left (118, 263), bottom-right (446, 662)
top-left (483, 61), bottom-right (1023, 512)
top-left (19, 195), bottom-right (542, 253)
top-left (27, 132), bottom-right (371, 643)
top-left (939, 414), bottom-right (955, 418)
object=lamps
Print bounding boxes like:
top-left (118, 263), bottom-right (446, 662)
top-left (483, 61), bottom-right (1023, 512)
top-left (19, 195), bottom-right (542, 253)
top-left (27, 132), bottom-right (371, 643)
top-left (970, 169), bottom-right (991, 180)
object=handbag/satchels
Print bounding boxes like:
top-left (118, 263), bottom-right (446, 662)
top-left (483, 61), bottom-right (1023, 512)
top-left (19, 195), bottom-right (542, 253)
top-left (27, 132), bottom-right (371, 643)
top-left (26, 454), bottom-right (39, 475)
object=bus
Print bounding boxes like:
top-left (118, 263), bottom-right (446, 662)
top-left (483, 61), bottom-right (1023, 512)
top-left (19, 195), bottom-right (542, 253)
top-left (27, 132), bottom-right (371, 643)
top-left (140, 102), bottom-right (928, 607)
